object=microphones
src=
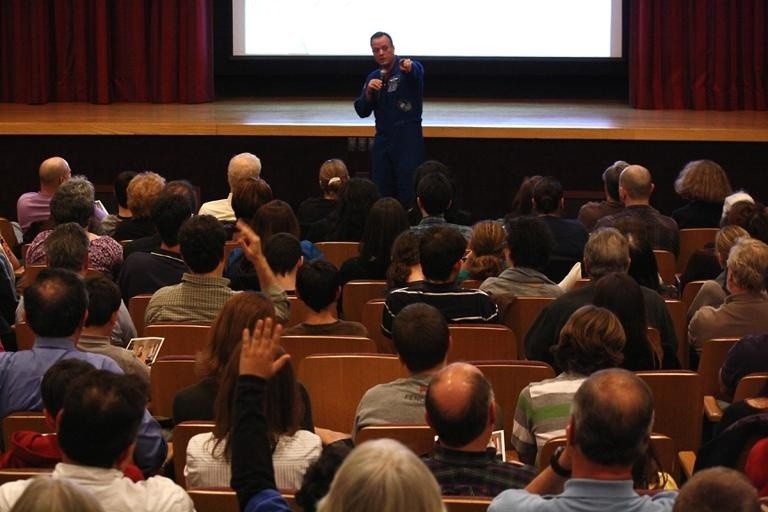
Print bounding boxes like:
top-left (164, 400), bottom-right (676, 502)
top-left (378, 69), bottom-right (388, 99)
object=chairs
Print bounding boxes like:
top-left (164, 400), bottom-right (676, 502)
top-left (1, 218), bottom-right (767, 512)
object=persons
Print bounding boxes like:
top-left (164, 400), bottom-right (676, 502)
top-left (1, 151), bottom-right (766, 512)
top-left (354, 32), bottom-right (426, 212)
